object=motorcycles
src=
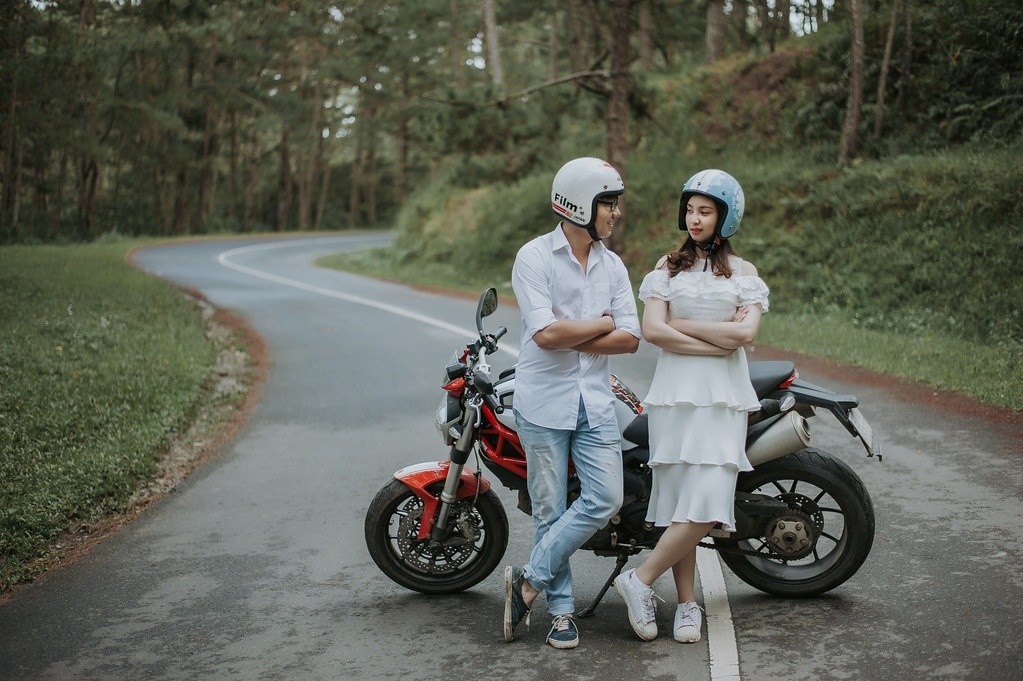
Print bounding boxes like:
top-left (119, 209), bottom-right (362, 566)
top-left (363, 311), bottom-right (884, 618)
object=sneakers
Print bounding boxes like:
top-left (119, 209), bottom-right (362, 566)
top-left (673, 602), bottom-right (705, 643)
top-left (545, 614), bottom-right (579, 649)
top-left (504, 566), bottom-right (533, 642)
top-left (614, 567), bottom-right (665, 642)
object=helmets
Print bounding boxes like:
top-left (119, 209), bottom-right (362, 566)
top-left (551, 157), bottom-right (625, 228)
top-left (677, 168), bottom-right (745, 238)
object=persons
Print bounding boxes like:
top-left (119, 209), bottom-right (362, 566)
top-left (614, 169), bottom-right (770, 643)
top-left (504, 157), bottom-right (641, 648)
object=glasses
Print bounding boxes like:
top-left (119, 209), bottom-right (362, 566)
top-left (596, 196), bottom-right (619, 211)
